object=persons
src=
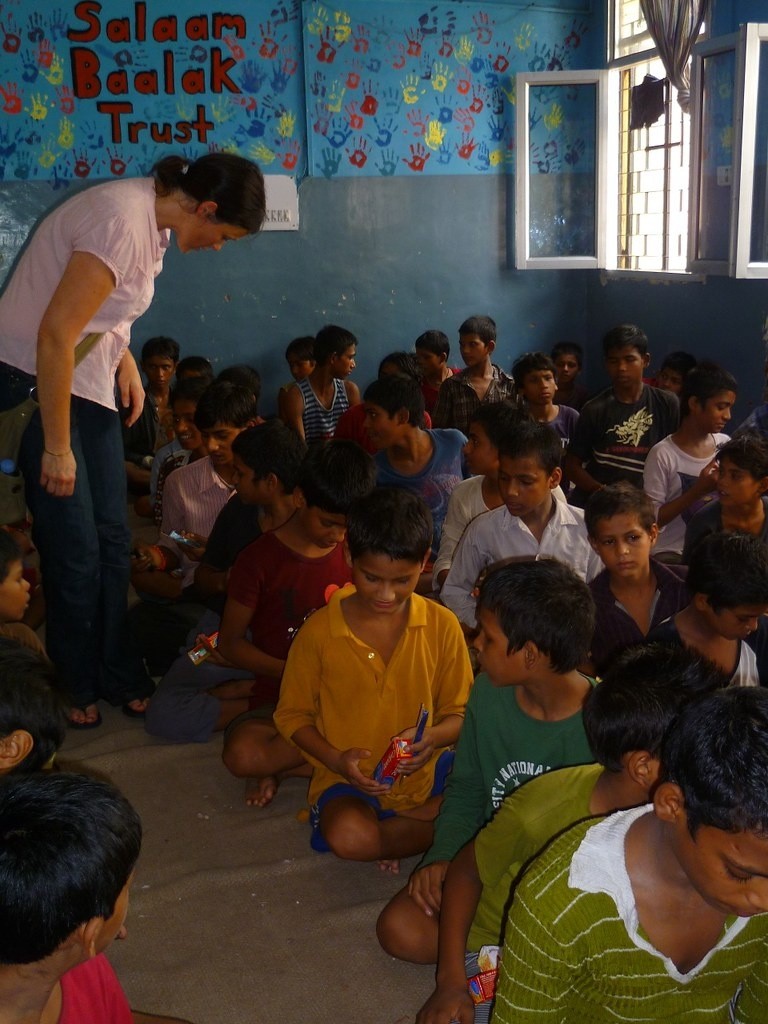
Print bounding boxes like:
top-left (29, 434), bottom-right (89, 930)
top-left (0, 152), bottom-right (268, 726)
top-left (0, 311), bottom-right (768, 1023)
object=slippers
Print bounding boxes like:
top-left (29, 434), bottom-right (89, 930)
top-left (61, 701), bottom-right (102, 730)
top-left (122, 686), bottom-right (156, 717)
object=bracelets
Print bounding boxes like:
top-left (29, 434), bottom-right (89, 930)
top-left (47, 450), bottom-right (72, 457)
